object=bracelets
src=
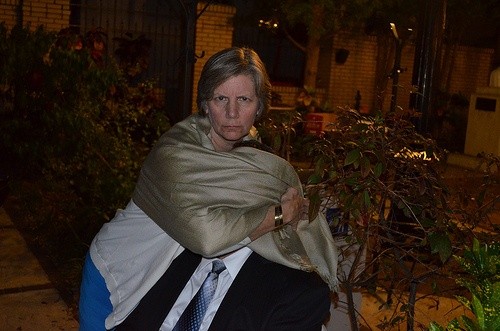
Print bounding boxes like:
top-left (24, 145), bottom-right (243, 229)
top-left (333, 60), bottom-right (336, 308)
top-left (274, 202), bottom-right (283, 232)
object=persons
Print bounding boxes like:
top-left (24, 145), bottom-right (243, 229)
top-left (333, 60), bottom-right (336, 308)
top-left (110, 139), bottom-right (332, 331)
top-left (79, 47), bottom-right (310, 331)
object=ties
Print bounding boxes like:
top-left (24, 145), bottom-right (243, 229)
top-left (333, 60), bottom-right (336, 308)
top-left (171, 260), bottom-right (227, 331)
